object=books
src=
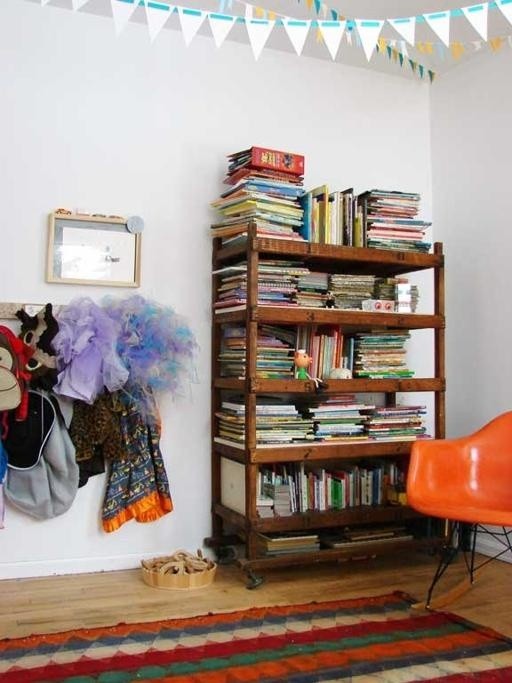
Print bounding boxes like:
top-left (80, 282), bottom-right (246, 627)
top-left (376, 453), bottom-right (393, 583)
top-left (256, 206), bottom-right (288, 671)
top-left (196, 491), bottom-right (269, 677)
top-left (213, 147), bottom-right (432, 253)
top-left (256, 526), bottom-right (415, 555)
top-left (213, 392), bottom-right (429, 444)
top-left (217, 321), bottom-right (416, 379)
top-left (219, 458), bottom-right (406, 519)
top-left (212, 259), bottom-right (419, 315)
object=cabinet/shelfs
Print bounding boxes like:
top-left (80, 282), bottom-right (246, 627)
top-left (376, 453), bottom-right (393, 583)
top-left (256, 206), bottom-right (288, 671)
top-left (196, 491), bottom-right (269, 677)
top-left (210, 226), bottom-right (448, 591)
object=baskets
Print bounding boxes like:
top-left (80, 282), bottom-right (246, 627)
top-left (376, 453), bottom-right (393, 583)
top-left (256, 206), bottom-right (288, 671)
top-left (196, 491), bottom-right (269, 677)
top-left (142, 554), bottom-right (218, 590)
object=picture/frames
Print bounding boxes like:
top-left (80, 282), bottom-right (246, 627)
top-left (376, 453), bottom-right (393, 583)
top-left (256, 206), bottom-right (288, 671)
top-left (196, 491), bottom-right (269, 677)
top-left (44, 213), bottom-right (142, 288)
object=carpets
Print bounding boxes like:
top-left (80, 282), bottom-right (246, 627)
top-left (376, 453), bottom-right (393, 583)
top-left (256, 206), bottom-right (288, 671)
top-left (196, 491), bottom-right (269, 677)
top-left (3, 589), bottom-right (511, 680)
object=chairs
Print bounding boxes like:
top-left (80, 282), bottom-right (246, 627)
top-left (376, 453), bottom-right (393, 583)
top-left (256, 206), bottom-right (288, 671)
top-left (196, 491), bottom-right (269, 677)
top-left (403, 411), bottom-right (511, 614)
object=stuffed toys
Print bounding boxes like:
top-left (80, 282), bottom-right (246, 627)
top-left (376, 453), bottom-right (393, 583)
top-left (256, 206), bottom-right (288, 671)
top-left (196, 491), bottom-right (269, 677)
top-left (0, 322), bottom-right (35, 423)
